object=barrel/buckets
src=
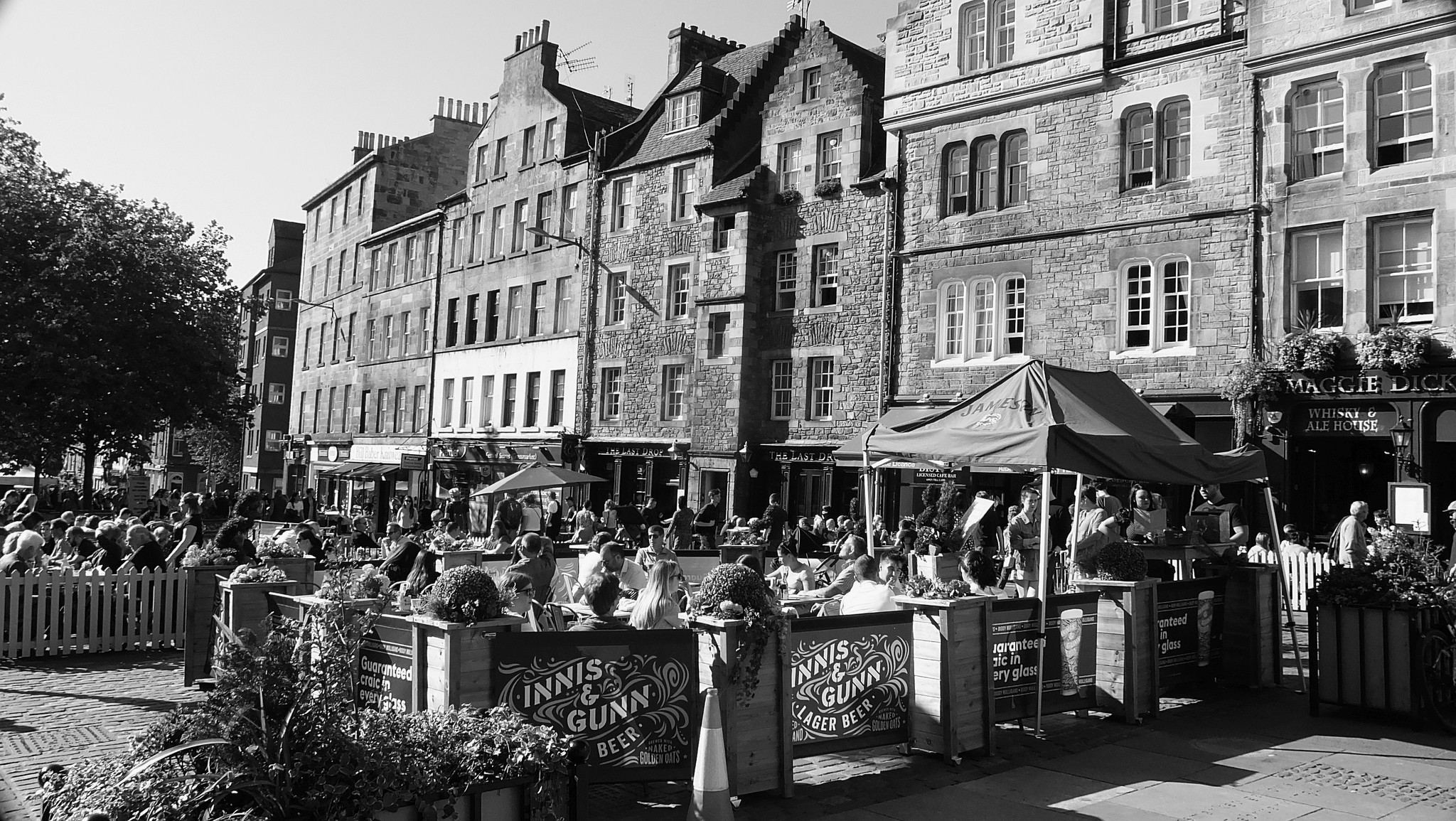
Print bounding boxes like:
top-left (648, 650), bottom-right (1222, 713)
top-left (769, 586), bottom-right (779, 598)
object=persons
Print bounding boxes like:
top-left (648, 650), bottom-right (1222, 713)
top-left (1442, 500), bottom-right (1456, 584)
top-left (1366, 510), bottom-right (1402, 542)
top-left (1327, 500), bottom-right (1371, 570)
top-left (0, 484), bottom-right (1307, 651)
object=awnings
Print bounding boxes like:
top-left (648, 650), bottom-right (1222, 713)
top-left (319, 461), bottom-right (401, 482)
top-left (829, 401), bottom-right (1184, 472)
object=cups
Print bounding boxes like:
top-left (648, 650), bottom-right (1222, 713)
top-left (411, 599), bottom-right (422, 613)
top-left (41, 556), bottom-right (50, 570)
top-left (370, 548), bottom-right (377, 558)
top-left (397, 596), bottom-right (411, 611)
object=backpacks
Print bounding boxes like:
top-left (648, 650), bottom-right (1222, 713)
top-left (690, 508), bottom-right (712, 535)
top-left (503, 499), bottom-right (521, 529)
top-left (451, 501), bottom-right (465, 531)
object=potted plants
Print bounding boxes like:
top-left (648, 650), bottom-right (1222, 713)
top-left (1276, 309), bottom-right (1345, 374)
top-left (1354, 304), bottom-right (1430, 376)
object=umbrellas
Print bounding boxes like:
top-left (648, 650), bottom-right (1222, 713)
top-left (469, 462), bottom-right (611, 537)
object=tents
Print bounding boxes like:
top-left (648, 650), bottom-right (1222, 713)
top-left (863, 356), bottom-right (1308, 737)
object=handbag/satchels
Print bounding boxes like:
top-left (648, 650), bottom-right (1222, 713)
top-left (540, 517), bottom-right (546, 532)
top-left (816, 599), bottom-right (841, 617)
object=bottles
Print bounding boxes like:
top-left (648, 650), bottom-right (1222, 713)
top-left (777, 588), bottom-right (781, 600)
top-left (782, 573), bottom-right (789, 600)
top-left (60, 552), bottom-right (69, 574)
top-left (1154, 533), bottom-right (1162, 544)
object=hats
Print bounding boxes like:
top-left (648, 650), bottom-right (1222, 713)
top-left (1442, 500), bottom-right (1456, 512)
top-left (448, 488), bottom-right (460, 497)
top-left (1027, 474), bottom-right (1043, 486)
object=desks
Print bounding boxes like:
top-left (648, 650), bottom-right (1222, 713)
top-left (1133, 542), bottom-right (1236, 580)
top-left (823, 544), bottom-right (841, 552)
top-left (545, 603), bottom-right (632, 623)
top-left (678, 582), bottom-right (701, 588)
top-left (778, 594), bottom-right (834, 607)
top-left (873, 545), bottom-right (901, 560)
top-left (46, 567), bottom-right (114, 592)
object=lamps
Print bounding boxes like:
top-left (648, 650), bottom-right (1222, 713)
top-left (574, 438), bottom-right (584, 457)
top-left (667, 441), bottom-right (680, 460)
top-left (1389, 415), bottom-right (1415, 476)
top-left (739, 441), bottom-right (753, 463)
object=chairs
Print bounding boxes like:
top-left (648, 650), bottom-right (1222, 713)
top-left (526, 576), bottom-right (579, 632)
top-left (561, 572), bottom-right (583, 603)
top-left (389, 581), bottom-right (435, 598)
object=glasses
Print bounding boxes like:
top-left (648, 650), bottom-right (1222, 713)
top-left (431, 519), bottom-right (438, 522)
top-left (404, 499), bottom-right (411, 503)
top-left (360, 522), bottom-right (366, 525)
top-left (648, 533), bottom-right (663, 539)
top-left (438, 525), bottom-right (446, 528)
top-left (619, 588), bottom-right (623, 597)
top-left (296, 538), bottom-right (304, 542)
top-left (601, 554), bottom-right (617, 565)
top-left (149, 503), bottom-right (153, 505)
top-left (672, 573), bottom-right (681, 581)
top-left (1195, 483), bottom-right (1209, 491)
top-left (519, 586), bottom-right (536, 596)
top-left (67, 537), bottom-right (71, 540)
top-left (416, 526), bottom-right (422, 531)
top-left (386, 531), bottom-right (395, 535)
top-left (1449, 511), bottom-right (1455, 516)
